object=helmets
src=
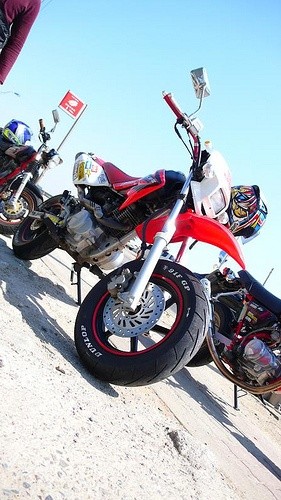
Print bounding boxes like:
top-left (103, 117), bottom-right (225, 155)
top-left (3, 118), bottom-right (33, 146)
top-left (227, 185), bottom-right (268, 245)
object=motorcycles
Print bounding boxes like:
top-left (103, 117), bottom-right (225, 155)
top-left (9, 64), bottom-right (267, 388)
top-left (184, 264), bottom-right (280, 414)
top-left (0, 107), bottom-right (65, 235)
